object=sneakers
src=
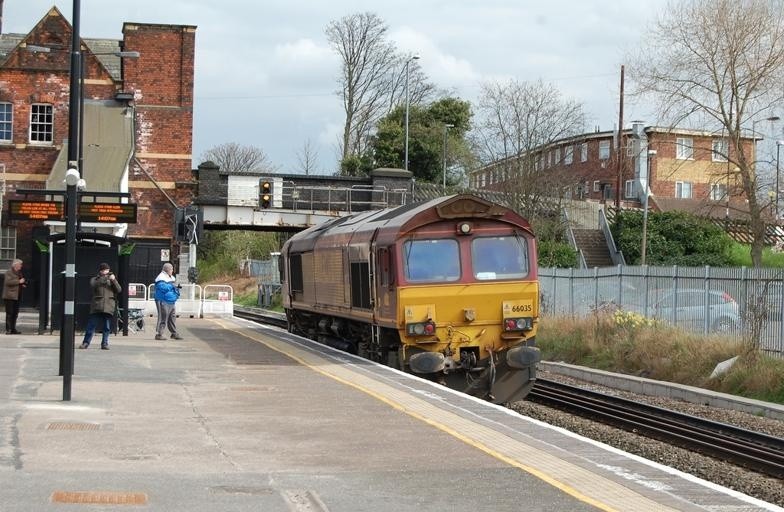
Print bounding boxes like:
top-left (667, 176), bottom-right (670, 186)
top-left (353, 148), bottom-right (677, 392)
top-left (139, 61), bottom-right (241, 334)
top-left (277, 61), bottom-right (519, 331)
top-left (101, 345), bottom-right (111, 350)
top-left (171, 335), bottom-right (183, 340)
top-left (5, 328), bottom-right (20, 335)
top-left (79, 342), bottom-right (87, 349)
top-left (156, 335), bottom-right (167, 341)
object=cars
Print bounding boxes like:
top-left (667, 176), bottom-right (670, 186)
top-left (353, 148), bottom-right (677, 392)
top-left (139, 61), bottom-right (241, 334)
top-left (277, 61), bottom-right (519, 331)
top-left (752, 282), bottom-right (782, 319)
top-left (621, 289), bottom-right (740, 337)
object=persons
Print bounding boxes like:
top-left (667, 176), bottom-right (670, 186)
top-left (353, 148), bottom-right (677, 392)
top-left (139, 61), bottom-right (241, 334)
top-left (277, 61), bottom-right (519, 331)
top-left (1, 258), bottom-right (28, 335)
top-left (76, 262), bottom-right (122, 350)
top-left (152, 261), bottom-right (183, 340)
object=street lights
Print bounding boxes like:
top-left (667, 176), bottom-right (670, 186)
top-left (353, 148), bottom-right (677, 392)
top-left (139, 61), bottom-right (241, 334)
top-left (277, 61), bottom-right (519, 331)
top-left (404, 55), bottom-right (421, 171)
top-left (19, 42), bottom-right (142, 243)
top-left (442, 124), bottom-right (455, 197)
top-left (775, 140), bottom-right (784, 218)
top-left (751, 116), bottom-right (779, 167)
top-left (640, 149), bottom-right (659, 264)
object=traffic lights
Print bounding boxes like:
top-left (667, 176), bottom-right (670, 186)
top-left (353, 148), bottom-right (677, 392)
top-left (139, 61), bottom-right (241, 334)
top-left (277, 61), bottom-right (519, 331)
top-left (257, 178), bottom-right (274, 210)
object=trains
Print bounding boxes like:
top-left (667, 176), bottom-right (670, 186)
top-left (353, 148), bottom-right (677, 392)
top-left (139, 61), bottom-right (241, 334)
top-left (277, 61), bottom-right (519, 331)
top-left (278, 193), bottom-right (544, 406)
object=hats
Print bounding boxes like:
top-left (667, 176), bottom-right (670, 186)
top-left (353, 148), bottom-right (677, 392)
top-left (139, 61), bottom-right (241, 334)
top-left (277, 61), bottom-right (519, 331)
top-left (98, 262), bottom-right (110, 270)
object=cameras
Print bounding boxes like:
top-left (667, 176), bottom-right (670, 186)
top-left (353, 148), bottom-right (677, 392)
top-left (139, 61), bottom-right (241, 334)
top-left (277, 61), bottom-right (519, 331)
top-left (178, 284), bottom-right (182, 288)
